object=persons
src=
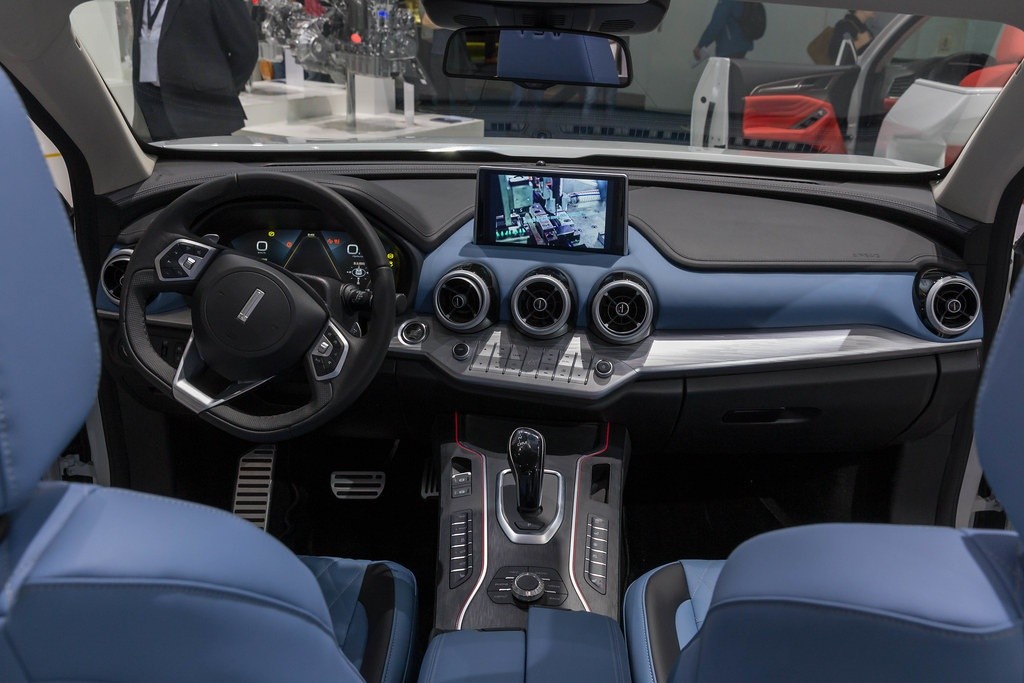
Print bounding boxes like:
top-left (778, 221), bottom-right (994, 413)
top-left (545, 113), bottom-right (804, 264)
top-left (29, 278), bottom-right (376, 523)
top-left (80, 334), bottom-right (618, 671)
top-left (830, 9), bottom-right (875, 66)
top-left (693, 0), bottom-right (753, 60)
top-left (130, 0), bottom-right (259, 142)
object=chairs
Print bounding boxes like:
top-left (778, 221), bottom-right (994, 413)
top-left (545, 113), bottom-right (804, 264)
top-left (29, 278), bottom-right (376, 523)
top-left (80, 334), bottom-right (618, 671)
top-left (0, 68), bottom-right (1024, 683)
top-left (499, 31), bottom-right (619, 84)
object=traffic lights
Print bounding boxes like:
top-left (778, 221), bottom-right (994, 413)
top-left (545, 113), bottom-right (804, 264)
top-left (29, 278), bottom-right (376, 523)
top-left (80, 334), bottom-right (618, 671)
top-left (346, 0), bottom-right (367, 44)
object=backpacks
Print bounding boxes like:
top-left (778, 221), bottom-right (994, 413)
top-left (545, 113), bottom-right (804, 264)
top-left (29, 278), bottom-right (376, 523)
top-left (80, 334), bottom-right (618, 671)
top-left (731, 1), bottom-right (766, 39)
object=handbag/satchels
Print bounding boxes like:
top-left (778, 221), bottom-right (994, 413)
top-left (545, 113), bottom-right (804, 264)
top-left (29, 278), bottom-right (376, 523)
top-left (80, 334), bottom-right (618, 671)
top-left (806, 20), bottom-right (861, 64)
